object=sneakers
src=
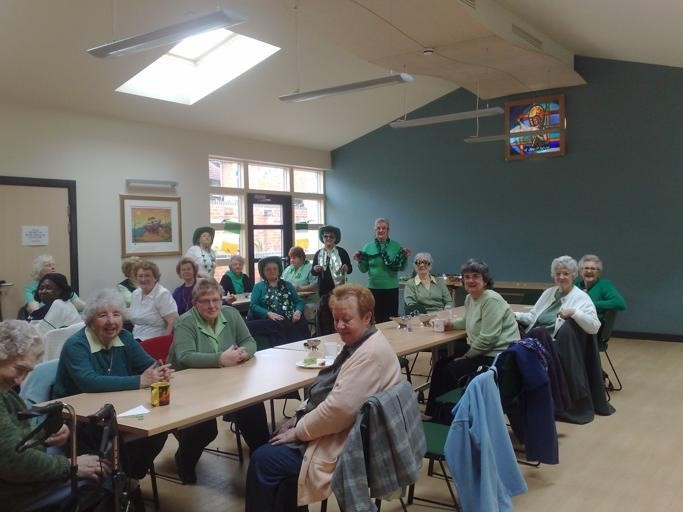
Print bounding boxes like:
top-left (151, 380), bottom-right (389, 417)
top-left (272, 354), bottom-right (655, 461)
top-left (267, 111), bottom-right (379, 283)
top-left (175, 448), bottom-right (195, 484)
top-left (422, 418), bottom-right (452, 426)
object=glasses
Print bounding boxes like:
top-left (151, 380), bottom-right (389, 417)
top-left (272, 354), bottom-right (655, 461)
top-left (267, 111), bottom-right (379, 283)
top-left (195, 299), bottom-right (223, 305)
top-left (324, 236), bottom-right (336, 239)
top-left (556, 271), bottom-right (571, 279)
top-left (463, 275), bottom-right (481, 280)
top-left (415, 260), bottom-right (430, 266)
top-left (583, 267), bottom-right (599, 272)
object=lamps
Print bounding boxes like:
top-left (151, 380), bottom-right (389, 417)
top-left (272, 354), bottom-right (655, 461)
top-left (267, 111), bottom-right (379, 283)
top-left (278, 11), bottom-right (412, 105)
top-left (464, 79), bottom-right (562, 143)
top-left (86, 1), bottom-right (248, 60)
top-left (388, 65), bottom-right (504, 130)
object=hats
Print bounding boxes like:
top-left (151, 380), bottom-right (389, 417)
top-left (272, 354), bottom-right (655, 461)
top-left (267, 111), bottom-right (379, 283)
top-left (258, 256), bottom-right (282, 281)
top-left (193, 227), bottom-right (215, 245)
top-left (318, 225), bottom-right (341, 244)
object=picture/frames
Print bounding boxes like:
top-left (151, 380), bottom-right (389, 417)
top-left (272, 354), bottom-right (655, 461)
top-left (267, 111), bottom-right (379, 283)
top-left (503, 93), bottom-right (566, 160)
top-left (120, 194), bottom-right (183, 257)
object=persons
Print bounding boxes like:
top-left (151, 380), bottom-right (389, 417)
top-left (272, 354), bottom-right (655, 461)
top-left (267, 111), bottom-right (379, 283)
top-left (245, 284), bottom-right (401, 512)
top-left (574, 254), bottom-right (627, 316)
top-left (1, 319), bottom-right (111, 511)
top-left (29, 274), bottom-right (84, 334)
top-left (512, 255), bottom-right (600, 344)
top-left (50, 288), bottom-right (174, 482)
top-left (424, 258), bottom-right (521, 418)
top-left (120, 225), bottom-right (352, 342)
top-left (166, 277), bottom-right (270, 485)
top-left (353, 219), bottom-right (411, 323)
top-left (405, 252), bottom-right (452, 314)
top-left (24, 254), bottom-right (87, 313)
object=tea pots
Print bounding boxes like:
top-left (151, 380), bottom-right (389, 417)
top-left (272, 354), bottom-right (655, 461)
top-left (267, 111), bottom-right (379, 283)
top-left (389, 315), bottom-right (411, 331)
top-left (414, 312), bottom-right (437, 328)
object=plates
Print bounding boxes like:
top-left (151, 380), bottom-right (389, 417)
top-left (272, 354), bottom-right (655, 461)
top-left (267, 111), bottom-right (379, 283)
top-left (294, 358), bottom-right (333, 370)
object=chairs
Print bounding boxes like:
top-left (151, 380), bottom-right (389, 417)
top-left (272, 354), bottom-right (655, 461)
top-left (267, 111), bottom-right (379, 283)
top-left (315, 291), bottom-right (412, 390)
top-left (21, 358), bottom-right (158, 510)
top-left (594, 309), bottom-right (623, 398)
top-left (434, 343), bottom-right (543, 465)
top-left (140, 335), bottom-right (242, 484)
top-left (409, 372), bottom-right (497, 509)
top-left (244, 319), bottom-right (278, 433)
top-left (320, 381), bottom-right (410, 511)
top-left (44, 326), bottom-right (79, 361)
top-left (24, 446), bottom-right (89, 511)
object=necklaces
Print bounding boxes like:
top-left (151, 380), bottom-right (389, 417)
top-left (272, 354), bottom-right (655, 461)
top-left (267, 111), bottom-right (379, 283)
top-left (99, 349), bottom-right (114, 375)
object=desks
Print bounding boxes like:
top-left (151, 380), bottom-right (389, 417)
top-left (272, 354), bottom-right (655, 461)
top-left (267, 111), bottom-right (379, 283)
top-left (445, 276), bottom-right (556, 307)
top-left (222, 289), bottom-right (317, 312)
top-left (275, 314), bottom-right (467, 402)
top-left (31, 347), bottom-right (337, 512)
top-left (428, 301), bottom-right (535, 328)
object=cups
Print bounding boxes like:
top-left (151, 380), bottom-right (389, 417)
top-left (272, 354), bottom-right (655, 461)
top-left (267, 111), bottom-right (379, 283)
top-left (433, 319), bottom-right (445, 333)
top-left (445, 302), bottom-right (453, 320)
top-left (324, 342), bottom-right (340, 356)
top-left (151, 382), bottom-right (169, 405)
